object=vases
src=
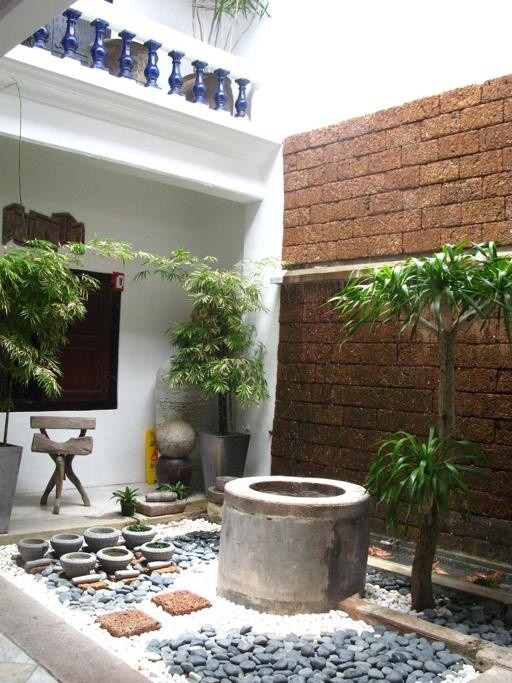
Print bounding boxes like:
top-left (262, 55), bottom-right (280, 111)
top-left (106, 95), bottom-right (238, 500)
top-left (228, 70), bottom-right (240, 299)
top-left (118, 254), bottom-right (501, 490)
top-left (16, 524), bottom-right (122, 580)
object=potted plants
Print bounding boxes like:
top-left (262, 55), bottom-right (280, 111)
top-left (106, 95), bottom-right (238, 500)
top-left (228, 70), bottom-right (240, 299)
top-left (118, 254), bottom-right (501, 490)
top-left (130, 246), bottom-right (268, 491)
top-left (97, 485), bottom-right (177, 577)
top-left (1, 234), bottom-right (101, 534)
